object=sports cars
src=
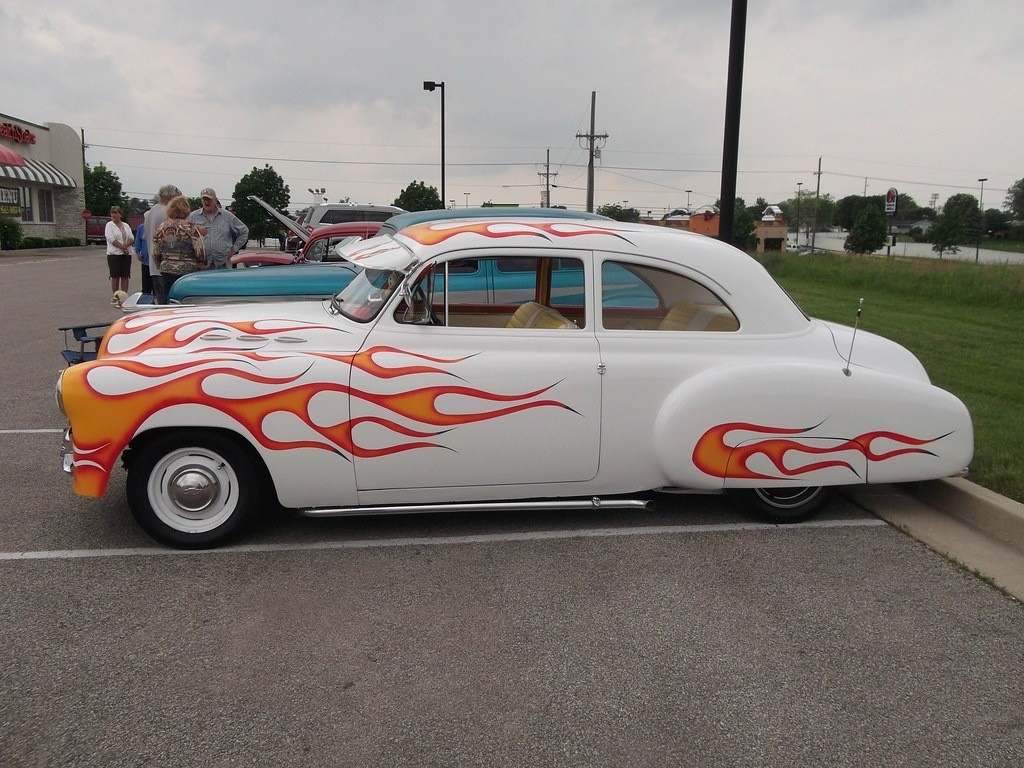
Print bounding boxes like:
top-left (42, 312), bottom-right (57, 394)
top-left (53, 215), bottom-right (978, 550)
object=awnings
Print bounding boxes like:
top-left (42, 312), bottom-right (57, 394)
top-left (0, 144), bottom-right (77, 191)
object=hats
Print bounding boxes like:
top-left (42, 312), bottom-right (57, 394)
top-left (201, 188), bottom-right (216, 200)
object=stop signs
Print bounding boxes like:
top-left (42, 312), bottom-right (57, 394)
top-left (82, 210), bottom-right (91, 219)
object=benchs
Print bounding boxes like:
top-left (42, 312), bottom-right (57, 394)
top-left (504, 301), bottom-right (581, 329)
top-left (657, 302), bottom-right (739, 332)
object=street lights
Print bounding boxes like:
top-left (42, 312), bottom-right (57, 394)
top-left (450, 199), bottom-right (455, 210)
top-left (420, 80), bottom-right (448, 211)
top-left (796, 181), bottom-right (804, 248)
top-left (463, 193), bottom-right (471, 208)
top-left (978, 177), bottom-right (990, 240)
top-left (686, 190), bottom-right (693, 210)
top-left (622, 201), bottom-right (629, 211)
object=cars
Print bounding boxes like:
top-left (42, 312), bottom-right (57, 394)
top-left (86, 216), bottom-right (113, 246)
top-left (161, 194), bottom-right (661, 313)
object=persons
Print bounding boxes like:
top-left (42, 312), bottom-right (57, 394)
top-left (105, 206), bottom-right (134, 296)
top-left (134, 185), bottom-right (249, 305)
